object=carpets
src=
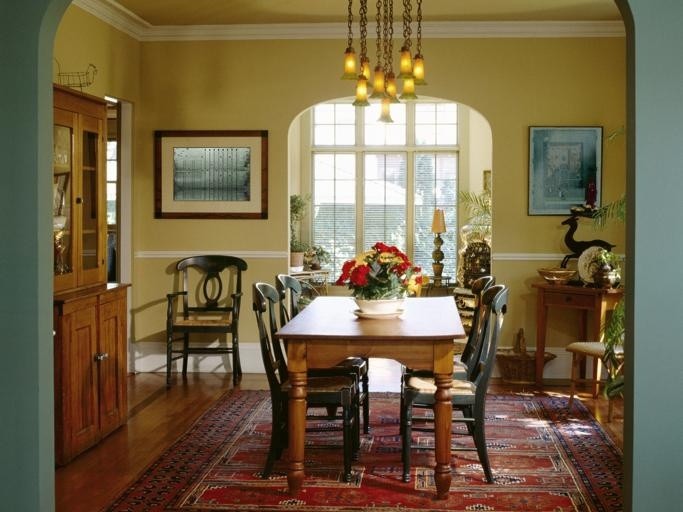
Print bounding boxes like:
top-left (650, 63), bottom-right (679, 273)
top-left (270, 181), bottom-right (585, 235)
top-left (101, 388), bottom-right (627, 511)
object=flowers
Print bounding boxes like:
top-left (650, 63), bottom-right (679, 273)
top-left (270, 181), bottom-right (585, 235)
top-left (332, 240), bottom-right (428, 297)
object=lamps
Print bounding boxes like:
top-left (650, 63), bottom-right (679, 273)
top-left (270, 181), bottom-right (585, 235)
top-left (342, 0), bottom-right (428, 124)
top-left (432, 209), bottom-right (448, 286)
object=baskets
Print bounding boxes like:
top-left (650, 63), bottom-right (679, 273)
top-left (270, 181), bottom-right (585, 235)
top-left (494, 329), bottom-right (556, 385)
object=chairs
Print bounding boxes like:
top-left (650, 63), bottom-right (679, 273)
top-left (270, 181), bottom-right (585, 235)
top-left (567, 292), bottom-right (625, 421)
top-left (399, 275), bottom-right (508, 485)
top-left (252, 273), bottom-right (370, 481)
top-left (167, 255), bottom-right (249, 386)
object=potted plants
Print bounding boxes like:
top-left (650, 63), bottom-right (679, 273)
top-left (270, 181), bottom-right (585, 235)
top-left (306, 245), bottom-right (332, 270)
top-left (290, 193), bottom-right (315, 266)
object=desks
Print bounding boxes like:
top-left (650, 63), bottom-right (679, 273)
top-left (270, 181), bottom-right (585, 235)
top-left (274, 294), bottom-right (468, 500)
top-left (303, 269), bottom-right (330, 296)
top-left (532, 282), bottom-right (625, 399)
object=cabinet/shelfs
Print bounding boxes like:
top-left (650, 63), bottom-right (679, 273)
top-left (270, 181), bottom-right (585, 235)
top-left (55, 282), bottom-right (132, 468)
top-left (52, 81), bottom-right (115, 303)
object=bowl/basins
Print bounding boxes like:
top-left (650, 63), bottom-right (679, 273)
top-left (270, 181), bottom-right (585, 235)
top-left (52, 215), bottom-right (67, 230)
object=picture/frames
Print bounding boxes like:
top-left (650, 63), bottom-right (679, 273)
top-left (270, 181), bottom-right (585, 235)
top-left (528, 125), bottom-right (602, 217)
top-left (153, 129), bottom-right (268, 220)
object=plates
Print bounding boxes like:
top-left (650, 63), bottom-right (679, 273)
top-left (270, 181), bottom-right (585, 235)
top-left (353, 308), bottom-right (403, 320)
top-left (576, 246), bottom-right (609, 281)
